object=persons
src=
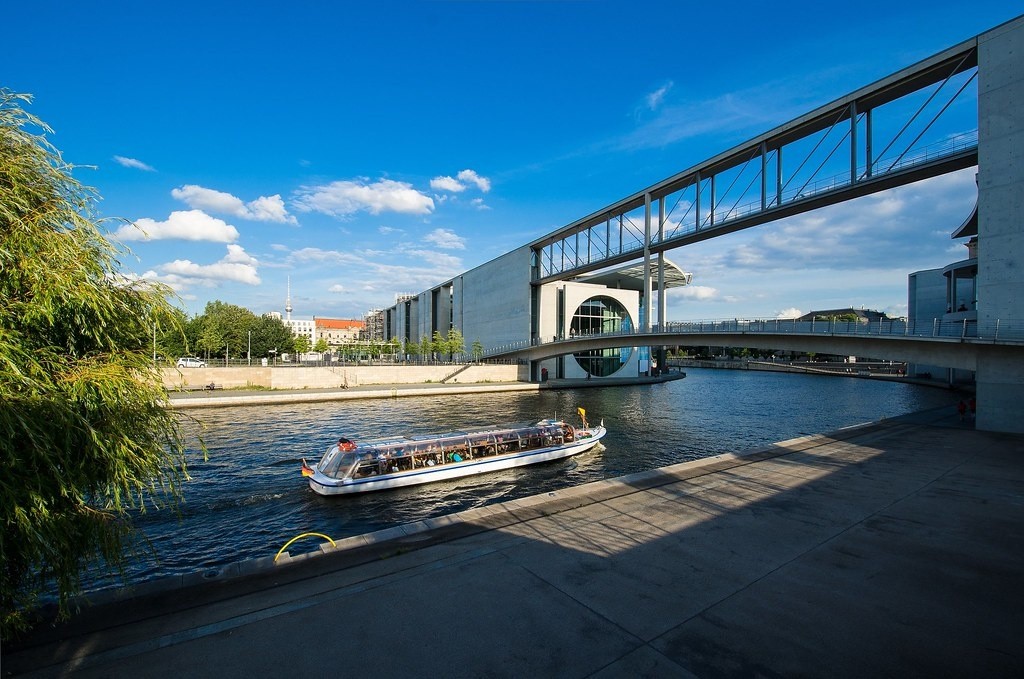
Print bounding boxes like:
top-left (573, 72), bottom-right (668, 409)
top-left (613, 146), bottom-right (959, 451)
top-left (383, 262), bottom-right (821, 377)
top-left (361, 425), bottom-right (576, 474)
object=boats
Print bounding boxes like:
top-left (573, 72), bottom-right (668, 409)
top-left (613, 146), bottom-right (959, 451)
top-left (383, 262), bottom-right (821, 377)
top-left (301, 408), bottom-right (607, 496)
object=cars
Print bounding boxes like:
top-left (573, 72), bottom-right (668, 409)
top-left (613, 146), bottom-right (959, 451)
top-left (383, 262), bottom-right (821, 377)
top-left (176, 358), bottom-right (209, 368)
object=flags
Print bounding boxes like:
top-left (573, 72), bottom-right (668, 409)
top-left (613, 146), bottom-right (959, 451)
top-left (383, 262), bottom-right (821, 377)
top-left (578, 407), bottom-right (586, 416)
top-left (600, 418), bottom-right (604, 427)
top-left (301, 458), bottom-right (315, 477)
